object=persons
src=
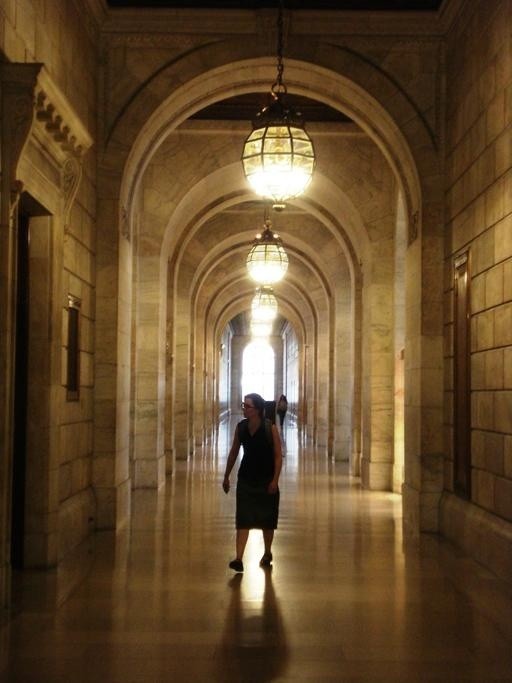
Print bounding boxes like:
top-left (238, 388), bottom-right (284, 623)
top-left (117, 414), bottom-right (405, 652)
top-left (221, 566), bottom-right (289, 683)
top-left (222, 393), bottom-right (283, 571)
top-left (277, 394), bottom-right (287, 431)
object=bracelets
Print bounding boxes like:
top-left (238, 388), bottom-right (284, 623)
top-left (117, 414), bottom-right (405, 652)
top-left (224, 474), bottom-right (230, 479)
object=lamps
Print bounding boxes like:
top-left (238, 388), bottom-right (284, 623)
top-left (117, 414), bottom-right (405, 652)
top-left (250, 287), bottom-right (281, 321)
top-left (245, 201), bottom-right (301, 291)
top-left (240, 1), bottom-right (318, 210)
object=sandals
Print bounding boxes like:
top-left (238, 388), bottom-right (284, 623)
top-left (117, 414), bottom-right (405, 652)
top-left (259, 553), bottom-right (272, 565)
top-left (228, 559), bottom-right (243, 572)
